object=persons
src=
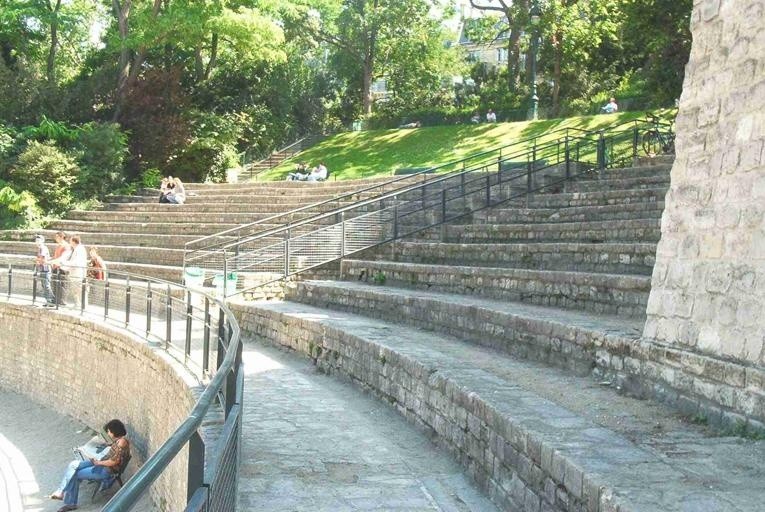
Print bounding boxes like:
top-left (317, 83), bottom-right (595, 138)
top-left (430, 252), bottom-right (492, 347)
top-left (286, 164), bottom-right (306, 181)
top-left (158, 176), bottom-right (185, 203)
top-left (487, 109), bottom-right (497, 123)
top-left (308, 162), bottom-right (327, 181)
top-left (33, 232), bottom-right (106, 310)
top-left (600, 98), bottom-right (618, 113)
top-left (50, 420), bottom-right (129, 512)
top-left (471, 112), bottom-right (481, 124)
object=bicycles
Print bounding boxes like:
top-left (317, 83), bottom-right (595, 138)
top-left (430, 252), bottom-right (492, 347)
top-left (642, 109), bottom-right (675, 156)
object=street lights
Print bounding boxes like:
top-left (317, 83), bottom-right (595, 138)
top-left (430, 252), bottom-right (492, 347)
top-left (528, 1), bottom-right (542, 120)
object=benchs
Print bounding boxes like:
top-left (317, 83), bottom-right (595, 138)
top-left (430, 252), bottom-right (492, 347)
top-left (498, 160), bottom-right (546, 169)
top-left (394, 168), bottom-right (434, 175)
top-left (292, 169), bottom-right (330, 181)
top-left (72, 446), bottom-right (132, 499)
